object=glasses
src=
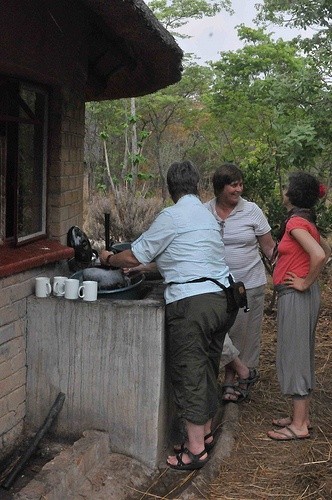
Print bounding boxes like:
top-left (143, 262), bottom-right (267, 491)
top-left (282, 184), bottom-right (289, 190)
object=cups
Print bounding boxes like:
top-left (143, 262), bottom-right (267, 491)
top-left (78, 281), bottom-right (98, 301)
top-left (35, 276), bottom-right (52, 297)
top-left (58, 278), bottom-right (79, 299)
top-left (53, 276), bottom-right (68, 296)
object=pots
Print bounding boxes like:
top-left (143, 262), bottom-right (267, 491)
top-left (112, 242), bottom-right (132, 253)
top-left (69, 269), bottom-right (146, 300)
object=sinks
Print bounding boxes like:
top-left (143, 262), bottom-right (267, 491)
top-left (98, 284), bottom-right (154, 302)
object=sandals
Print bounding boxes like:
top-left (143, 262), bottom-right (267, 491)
top-left (237, 367), bottom-right (259, 403)
top-left (174, 433), bottom-right (216, 452)
top-left (221, 384), bottom-right (238, 402)
top-left (166, 448), bottom-right (207, 469)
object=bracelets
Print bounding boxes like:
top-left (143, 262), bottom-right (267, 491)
top-left (105, 254), bottom-right (113, 267)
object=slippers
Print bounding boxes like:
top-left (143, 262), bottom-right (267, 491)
top-left (273, 416), bottom-right (312, 430)
top-left (266, 425), bottom-right (310, 442)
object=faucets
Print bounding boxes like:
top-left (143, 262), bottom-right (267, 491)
top-left (91, 250), bottom-right (98, 262)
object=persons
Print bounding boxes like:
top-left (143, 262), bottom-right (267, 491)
top-left (201, 163), bottom-right (274, 403)
top-left (100, 160), bottom-right (238, 471)
top-left (266, 173), bottom-right (331, 440)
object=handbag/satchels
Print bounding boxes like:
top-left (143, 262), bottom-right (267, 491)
top-left (226, 281), bottom-right (248, 313)
